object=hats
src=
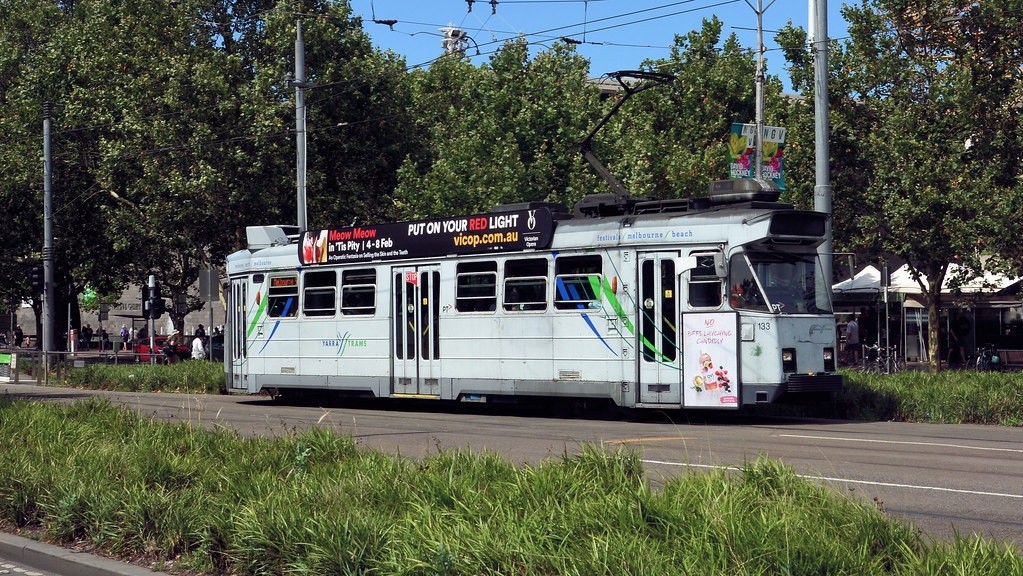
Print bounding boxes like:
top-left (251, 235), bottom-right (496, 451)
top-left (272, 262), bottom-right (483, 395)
top-left (172, 329), bottom-right (180, 335)
top-left (122, 324), bottom-right (127, 328)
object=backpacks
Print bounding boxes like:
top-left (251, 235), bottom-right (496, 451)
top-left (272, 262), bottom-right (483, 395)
top-left (122, 328), bottom-right (129, 337)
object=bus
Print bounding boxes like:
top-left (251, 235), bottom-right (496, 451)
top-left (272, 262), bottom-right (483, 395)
top-left (224, 71), bottom-right (856, 423)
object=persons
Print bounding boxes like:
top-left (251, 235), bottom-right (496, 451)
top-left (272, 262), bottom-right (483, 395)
top-left (944, 311), bottom-right (969, 367)
top-left (716, 267), bottom-right (750, 310)
top-left (836, 304), bottom-right (879, 366)
top-left (320, 286), bottom-right (531, 317)
top-left (0, 324), bottom-right (223, 366)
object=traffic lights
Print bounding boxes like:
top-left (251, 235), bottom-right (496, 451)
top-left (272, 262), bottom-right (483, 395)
top-left (32, 265), bottom-right (46, 290)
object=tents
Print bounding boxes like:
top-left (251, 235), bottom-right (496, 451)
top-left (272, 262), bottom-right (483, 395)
top-left (830, 262), bottom-right (1023, 363)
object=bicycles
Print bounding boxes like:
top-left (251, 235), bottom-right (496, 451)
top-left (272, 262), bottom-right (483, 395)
top-left (853, 344), bottom-right (906, 377)
top-left (964, 342), bottom-right (1001, 372)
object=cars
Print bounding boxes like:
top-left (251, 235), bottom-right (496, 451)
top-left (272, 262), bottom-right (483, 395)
top-left (133, 335), bottom-right (191, 366)
